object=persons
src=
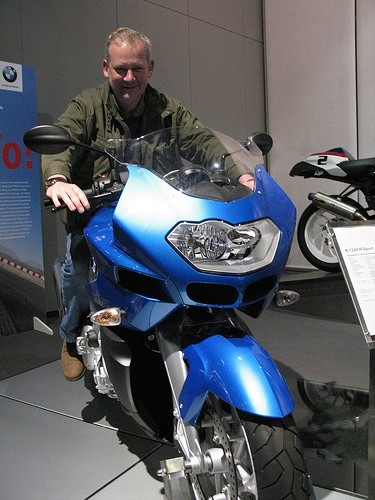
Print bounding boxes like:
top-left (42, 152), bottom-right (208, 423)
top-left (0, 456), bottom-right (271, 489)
top-left (41, 27), bottom-right (254, 381)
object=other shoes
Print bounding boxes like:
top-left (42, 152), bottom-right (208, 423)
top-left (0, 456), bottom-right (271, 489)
top-left (61, 337), bottom-right (87, 381)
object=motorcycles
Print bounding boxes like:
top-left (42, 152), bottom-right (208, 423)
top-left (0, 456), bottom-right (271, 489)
top-left (288, 146), bottom-right (375, 274)
top-left (22, 123), bottom-right (316, 500)
top-left (294, 377), bottom-right (370, 466)
